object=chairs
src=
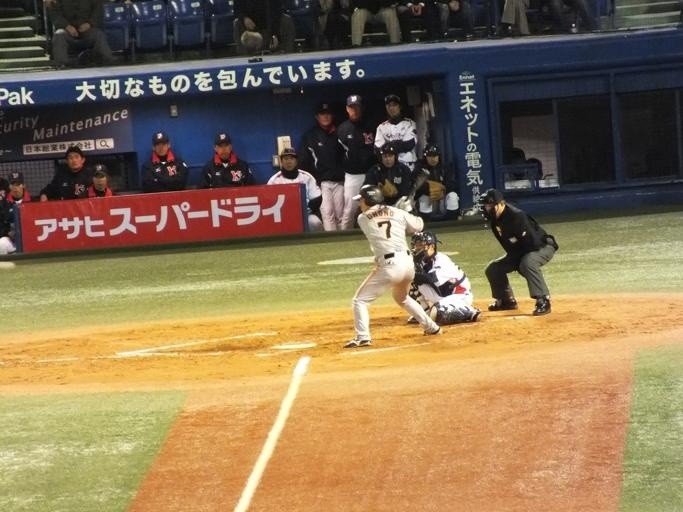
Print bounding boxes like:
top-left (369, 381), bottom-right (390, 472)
top-left (499, 148), bottom-right (545, 190)
top-left (69, 0), bottom-right (517, 66)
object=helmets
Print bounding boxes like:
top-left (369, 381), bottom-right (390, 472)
top-left (351, 184), bottom-right (385, 206)
top-left (478, 188), bottom-right (504, 204)
top-left (410, 230), bottom-right (438, 250)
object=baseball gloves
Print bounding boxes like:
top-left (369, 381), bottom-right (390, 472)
top-left (378, 179), bottom-right (396, 197)
top-left (426, 180), bottom-right (445, 201)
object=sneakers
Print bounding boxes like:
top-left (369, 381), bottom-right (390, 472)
top-left (423, 322), bottom-right (442, 336)
top-left (471, 308), bottom-right (482, 323)
top-left (407, 305), bottom-right (431, 324)
top-left (532, 294), bottom-right (552, 316)
top-left (343, 335), bottom-right (373, 348)
top-left (488, 296), bottom-right (517, 311)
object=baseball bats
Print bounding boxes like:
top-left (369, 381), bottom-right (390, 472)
top-left (405, 169), bottom-right (430, 199)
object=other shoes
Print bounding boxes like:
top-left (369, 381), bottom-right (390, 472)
top-left (269, 15), bottom-right (595, 56)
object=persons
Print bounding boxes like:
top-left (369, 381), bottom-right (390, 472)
top-left (346, 184), bottom-right (439, 348)
top-left (477, 188), bottom-right (558, 316)
top-left (403, 233), bottom-right (481, 325)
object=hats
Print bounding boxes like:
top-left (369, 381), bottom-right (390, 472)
top-left (379, 141), bottom-right (400, 155)
top-left (422, 143), bottom-right (442, 157)
top-left (152, 131), bottom-right (170, 145)
top-left (280, 147), bottom-right (297, 158)
top-left (91, 163), bottom-right (110, 178)
top-left (0, 177), bottom-right (11, 193)
top-left (345, 93), bottom-right (364, 108)
top-left (213, 130), bottom-right (232, 145)
top-left (7, 171), bottom-right (24, 184)
top-left (315, 102), bottom-right (334, 116)
top-left (383, 93), bottom-right (403, 108)
